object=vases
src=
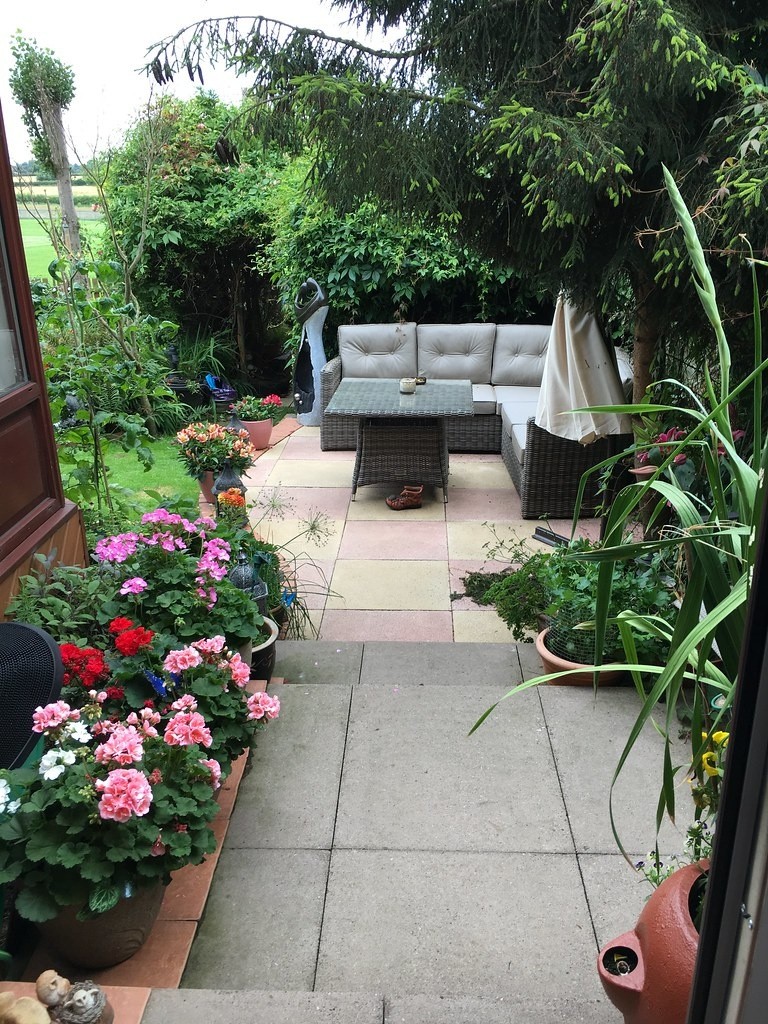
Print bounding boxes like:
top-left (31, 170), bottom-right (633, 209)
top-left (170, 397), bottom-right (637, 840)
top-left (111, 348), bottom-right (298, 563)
top-left (239, 638), bottom-right (252, 668)
top-left (249, 616), bottom-right (279, 684)
top-left (40, 876), bottom-right (166, 971)
top-left (596, 858), bottom-right (710, 1024)
top-left (239, 417), bottom-right (273, 449)
top-left (198, 470), bottom-right (222, 504)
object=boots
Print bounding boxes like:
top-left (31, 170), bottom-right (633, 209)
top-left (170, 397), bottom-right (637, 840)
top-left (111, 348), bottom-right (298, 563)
top-left (384, 485), bottom-right (423, 510)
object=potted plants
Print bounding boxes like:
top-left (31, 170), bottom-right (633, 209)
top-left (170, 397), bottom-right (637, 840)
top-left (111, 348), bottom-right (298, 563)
top-left (448, 515), bottom-right (676, 688)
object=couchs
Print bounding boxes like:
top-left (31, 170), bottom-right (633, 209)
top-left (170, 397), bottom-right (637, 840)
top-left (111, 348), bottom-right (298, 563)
top-left (320, 322), bottom-right (634, 521)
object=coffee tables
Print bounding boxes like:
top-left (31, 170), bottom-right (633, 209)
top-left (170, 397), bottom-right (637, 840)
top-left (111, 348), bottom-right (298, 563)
top-left (325, 376), bottom-right (474, 507)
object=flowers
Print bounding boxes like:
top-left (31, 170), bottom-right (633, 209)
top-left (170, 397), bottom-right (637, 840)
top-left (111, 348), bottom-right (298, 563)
top-left (635, 731), bottom-right (731, 919)
top-left (0, 485), bottom-right (346, 931)
top-left (225, 395), bottom-right (283, 421)
top-left (168, 420), bottom-right (256, 483)
